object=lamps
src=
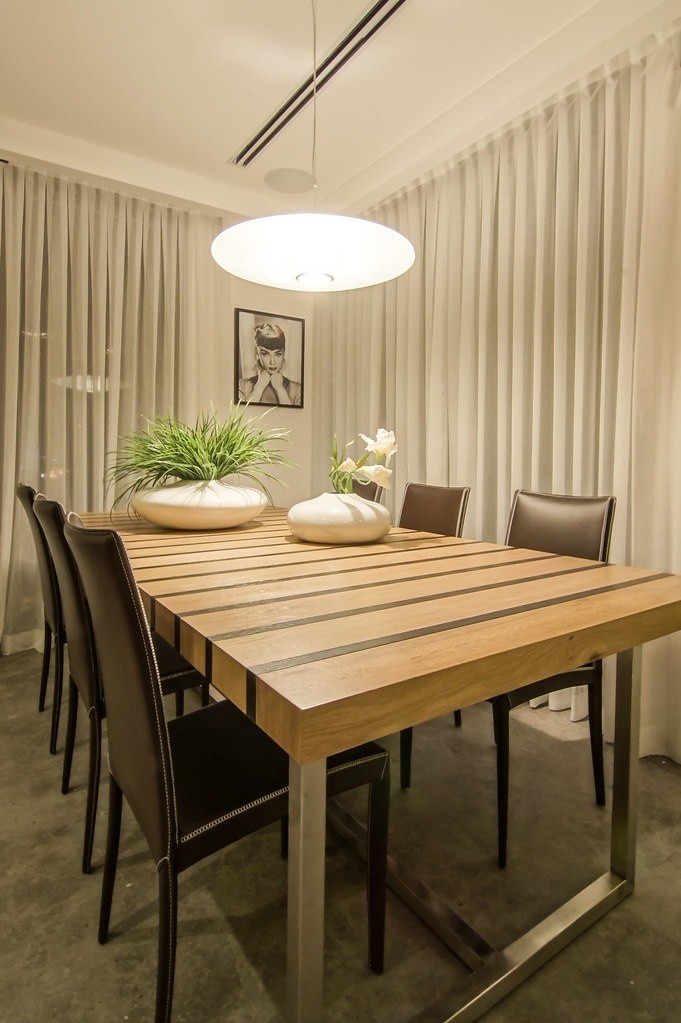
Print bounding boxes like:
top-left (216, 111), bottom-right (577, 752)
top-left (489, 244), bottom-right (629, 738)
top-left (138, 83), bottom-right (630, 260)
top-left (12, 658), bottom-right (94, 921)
top-left (211, 1), bottom-right (416, 293)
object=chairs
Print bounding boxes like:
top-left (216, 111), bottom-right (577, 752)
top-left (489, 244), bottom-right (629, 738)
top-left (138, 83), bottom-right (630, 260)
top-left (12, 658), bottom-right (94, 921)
top-left (30, 494), bottom-right (208, 875)
top-left (63, 511), bottom-right (394, 1019)
top-left (341, 479), bottom-right (384, 503)
top-left (395, 488), bottom-right (618, 871)
top-left (397, 481), bottom-right (472, 539)
top-left (15, 484), bottom-right (69, 755)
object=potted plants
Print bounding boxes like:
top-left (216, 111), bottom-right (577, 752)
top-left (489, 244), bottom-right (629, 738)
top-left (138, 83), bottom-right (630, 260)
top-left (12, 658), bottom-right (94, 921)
top-left (104, 396), bottom-right (295, 530)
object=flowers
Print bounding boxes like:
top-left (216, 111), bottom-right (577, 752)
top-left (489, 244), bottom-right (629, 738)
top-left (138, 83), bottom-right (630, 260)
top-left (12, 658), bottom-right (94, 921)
top-left (328, 427), bottom-right (399, 495)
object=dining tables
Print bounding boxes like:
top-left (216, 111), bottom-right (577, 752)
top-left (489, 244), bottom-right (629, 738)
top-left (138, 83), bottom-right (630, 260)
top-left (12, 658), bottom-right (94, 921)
top-left (75, 504), bottom-right (680, 1022)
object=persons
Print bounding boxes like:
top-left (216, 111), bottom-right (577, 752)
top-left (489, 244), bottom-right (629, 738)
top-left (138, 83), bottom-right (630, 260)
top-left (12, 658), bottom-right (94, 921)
top-left (238, 323), bottom-right (302, 406)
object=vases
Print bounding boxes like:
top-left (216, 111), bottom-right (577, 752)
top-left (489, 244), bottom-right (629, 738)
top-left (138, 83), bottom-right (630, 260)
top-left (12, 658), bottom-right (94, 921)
top-left (287, 491), bottom-right (392, 544)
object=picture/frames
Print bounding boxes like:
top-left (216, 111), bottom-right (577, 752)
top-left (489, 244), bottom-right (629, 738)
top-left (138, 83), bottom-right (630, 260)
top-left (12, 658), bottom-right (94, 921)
top-left (233, 307), bottom-right (305, 409)
